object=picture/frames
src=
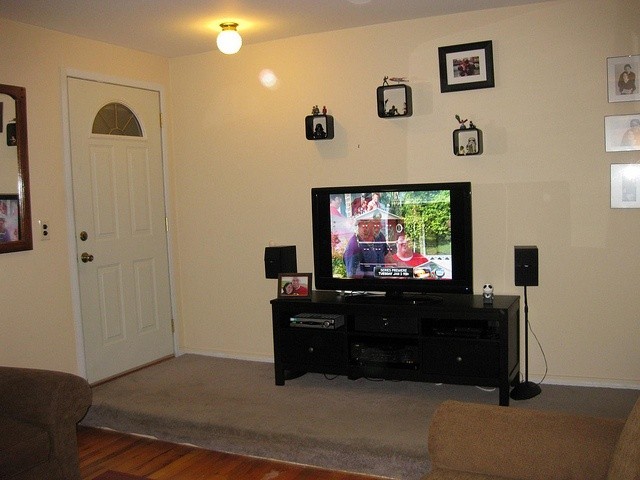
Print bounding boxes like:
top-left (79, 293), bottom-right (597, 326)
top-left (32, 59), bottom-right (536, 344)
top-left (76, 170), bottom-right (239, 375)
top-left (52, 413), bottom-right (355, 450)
top-left (6, 123), bottom-right (18, 146)
top-left (604, 113), bottom-right (640, 154)
top-left (277, 272), bottom-right (311, 300)
top-left (606, 54), bottom-right (640, 104)
top-left (0, 194), bottom-right (20, 241)
top-left (438, 40), bottom-right (495, 93)
top-left (609, 163), bottom-right (640, 209)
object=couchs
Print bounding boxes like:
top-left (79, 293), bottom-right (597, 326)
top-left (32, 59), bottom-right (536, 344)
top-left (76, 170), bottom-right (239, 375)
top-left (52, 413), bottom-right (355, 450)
top-left (422, 398), bottom-right (640, 480)
top-left (0, 366), bottom-right (93, 479)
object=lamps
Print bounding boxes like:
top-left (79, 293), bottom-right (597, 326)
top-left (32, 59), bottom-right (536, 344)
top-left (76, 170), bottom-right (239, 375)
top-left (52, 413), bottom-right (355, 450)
top-left (217, 23), bottom-right (244, 55)
top-left (0, 84), bottom-right (33, 254)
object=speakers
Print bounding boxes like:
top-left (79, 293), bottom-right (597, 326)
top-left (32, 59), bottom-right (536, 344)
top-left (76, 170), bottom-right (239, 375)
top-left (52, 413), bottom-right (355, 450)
top-left (514, 246), bottom-right (540, 288)
top-left (262, 245), bottom-right (298, 278)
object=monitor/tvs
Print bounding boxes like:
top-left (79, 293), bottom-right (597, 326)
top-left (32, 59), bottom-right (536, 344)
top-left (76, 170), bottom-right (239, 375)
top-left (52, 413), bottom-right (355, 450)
top-left (311, 181), bottom-right (474, 293)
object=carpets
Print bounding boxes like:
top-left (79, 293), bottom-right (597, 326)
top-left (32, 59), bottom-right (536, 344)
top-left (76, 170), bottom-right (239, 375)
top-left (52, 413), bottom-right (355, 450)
top-left (88, 352), bottom-right (640, 477)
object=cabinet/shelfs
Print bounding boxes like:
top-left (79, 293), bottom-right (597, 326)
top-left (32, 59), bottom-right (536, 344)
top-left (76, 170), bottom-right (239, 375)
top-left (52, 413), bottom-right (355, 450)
top-left (376, 84), bottom-right (412, 118)
top-left (452, 127), bottom-right (483, 156)
top-left (270, 294), bottom-right (520, 406)
top-left (305, 113), bottom-right (334, 140)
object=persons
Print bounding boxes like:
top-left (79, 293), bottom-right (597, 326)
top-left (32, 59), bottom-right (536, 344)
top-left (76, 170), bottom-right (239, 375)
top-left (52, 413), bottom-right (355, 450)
top-left (619, 119), bottom-right (640, 145)
top-left (463, 58), bottom-right (475, 76)
top-left (282, 282), bottom-right (295, 295)
top-left (619, 73), bottom-right (637, 95)
top-left (626, 128), bottom-right (635, 141)
top-left (10, 227), bottom-right (19, 241)
top-left (456, 64), bottom-right (466, 76)
top-left (313, 123), bottom-right (326, 138)
top-left (620, 63), bottom-right (636, 86)
top-left (0, 217), bottom-right (10, 242)
top-left (388, 105), bottom-right (398, 116)
top-left (293, 277), bottom-right (309, 296)
